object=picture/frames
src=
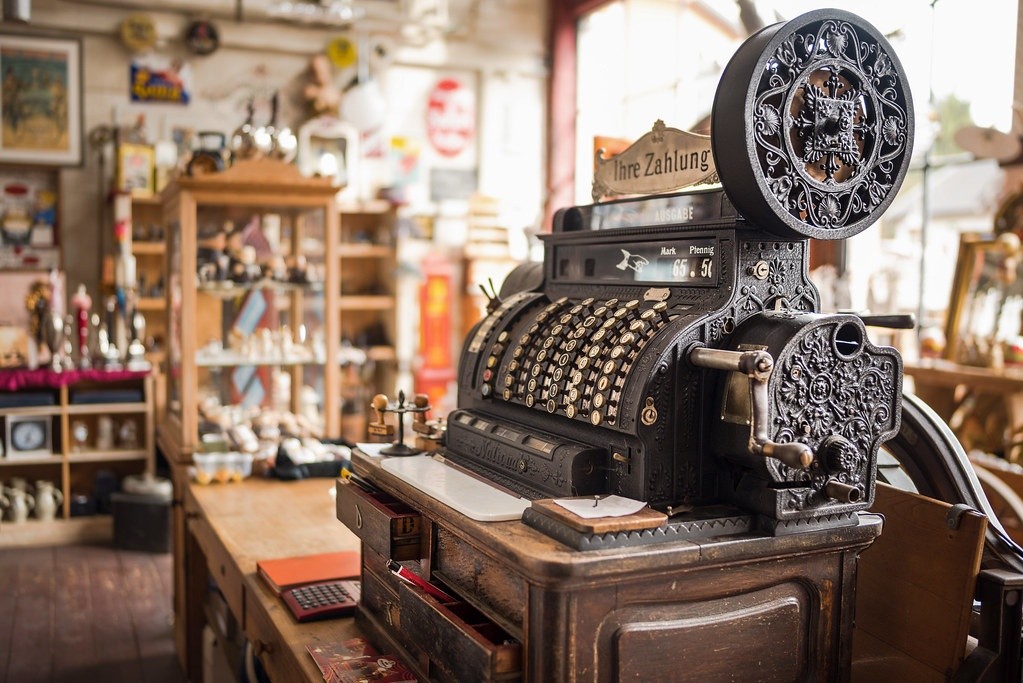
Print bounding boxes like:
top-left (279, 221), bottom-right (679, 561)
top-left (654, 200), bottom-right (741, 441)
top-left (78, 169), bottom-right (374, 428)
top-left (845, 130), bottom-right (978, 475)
top-left (0, 23), bottom-right (85, 167)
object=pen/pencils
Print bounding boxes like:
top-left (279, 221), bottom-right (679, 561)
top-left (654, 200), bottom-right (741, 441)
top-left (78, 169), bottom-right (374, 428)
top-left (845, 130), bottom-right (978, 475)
top-left (345, 475), bottom-right (391, 501)
top-left (384, 560), bottom-right (462, 605)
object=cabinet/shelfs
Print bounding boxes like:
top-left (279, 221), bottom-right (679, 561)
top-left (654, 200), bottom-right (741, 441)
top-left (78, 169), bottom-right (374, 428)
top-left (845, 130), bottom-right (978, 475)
top-left (156, 155), bottom-right (348, 478)
top-left (100, 190), bottom-right (167, 407)
top-left (0, 367), bottom-right (159, 547)
top-left (303, 198), bottom-right (414, 446)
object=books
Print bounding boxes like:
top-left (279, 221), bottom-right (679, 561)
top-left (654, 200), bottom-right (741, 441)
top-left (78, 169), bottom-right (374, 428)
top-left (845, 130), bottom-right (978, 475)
top-left (255, 549), bottom-right (365, 596)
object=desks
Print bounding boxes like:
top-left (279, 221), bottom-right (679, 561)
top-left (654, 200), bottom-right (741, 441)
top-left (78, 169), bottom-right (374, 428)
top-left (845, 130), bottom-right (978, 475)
top-left (173, 475), bottom-right (364, 683)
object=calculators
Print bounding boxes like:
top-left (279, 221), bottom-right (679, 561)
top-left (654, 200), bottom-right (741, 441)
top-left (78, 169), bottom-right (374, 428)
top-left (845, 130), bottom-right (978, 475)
top-left (283, 580), bottom-right (364, 624)
